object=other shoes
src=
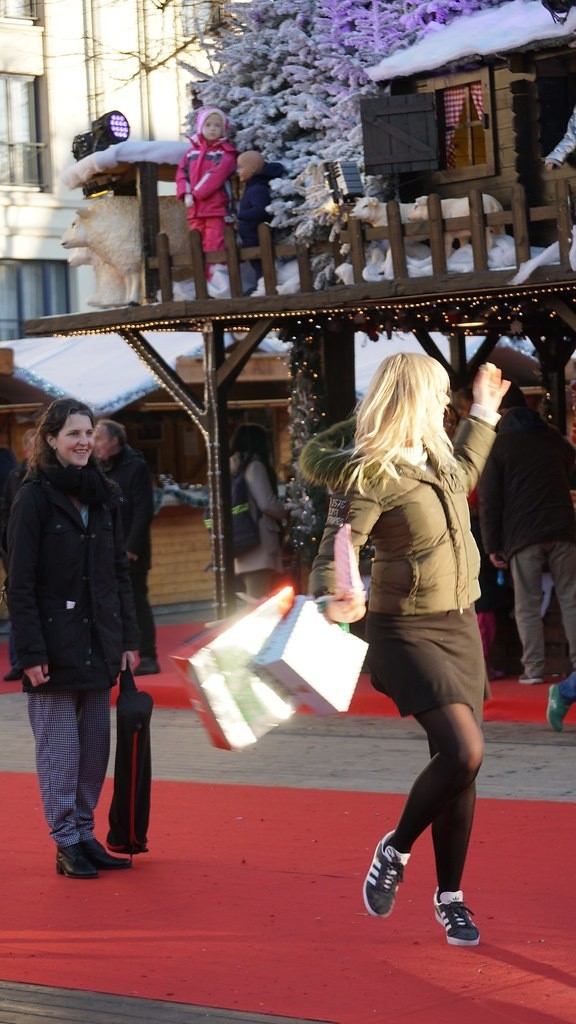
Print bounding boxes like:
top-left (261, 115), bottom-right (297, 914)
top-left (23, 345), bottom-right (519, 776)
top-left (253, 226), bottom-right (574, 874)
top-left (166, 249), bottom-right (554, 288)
top-left (133, 655), bottom-right (159, 675)
top-left (4, 667), bottom-right (24, 680)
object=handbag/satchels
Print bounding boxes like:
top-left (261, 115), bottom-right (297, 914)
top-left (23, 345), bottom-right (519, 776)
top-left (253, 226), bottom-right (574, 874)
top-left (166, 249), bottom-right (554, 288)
top-left (165, 581), bottom-right (371, 752)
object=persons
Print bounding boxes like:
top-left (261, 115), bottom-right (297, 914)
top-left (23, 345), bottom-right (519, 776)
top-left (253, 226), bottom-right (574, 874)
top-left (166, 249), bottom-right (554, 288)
top-left (230, 422), bottom-right (290, 598)
top-left (299, 352), bottom-right (511, 947)
top-left (444, 386), bottom-right (576, 731)
top-left (0, 429), bottom-right (36, 681)
top-left (176, 106), bottom-right (284, 283)
top-left (545, 108), bottom-right (576, 171)
top-left (7, 399), bottom-right (137, 877)
top-left (95, 422), bottom-right (159, 675)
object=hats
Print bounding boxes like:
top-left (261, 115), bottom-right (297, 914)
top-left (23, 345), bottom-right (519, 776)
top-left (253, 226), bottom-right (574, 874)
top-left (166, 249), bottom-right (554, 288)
top-left (196, 104), bottom-right (230, 137)
top-left (499, 384), bottom-right (529, 410)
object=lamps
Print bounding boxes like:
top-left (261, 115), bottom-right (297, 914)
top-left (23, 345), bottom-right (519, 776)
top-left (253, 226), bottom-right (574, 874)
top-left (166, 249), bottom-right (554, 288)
top-left (70, 110), bottom-right (131, 198)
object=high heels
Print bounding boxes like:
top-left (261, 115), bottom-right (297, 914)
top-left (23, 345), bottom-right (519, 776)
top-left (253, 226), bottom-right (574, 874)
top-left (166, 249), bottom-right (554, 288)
top-left (80, 840), bottom-right (131, 870)
top-left (56, 840), bottom-right (100, 879)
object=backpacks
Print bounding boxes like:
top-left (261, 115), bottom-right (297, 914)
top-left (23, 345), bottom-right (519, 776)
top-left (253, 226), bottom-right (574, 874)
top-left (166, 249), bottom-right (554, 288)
top-left (203, 458), bottom-right (262, 557)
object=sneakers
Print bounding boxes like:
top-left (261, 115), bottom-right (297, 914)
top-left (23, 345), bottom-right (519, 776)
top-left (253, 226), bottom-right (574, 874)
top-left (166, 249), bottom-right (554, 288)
top-left (545, 684), bottom-right (572, 733)
top-left (361, 830), bottom-right (413, 921)
top-left (432, 885), bottom-right (481, 946)
top-left (517, 672), bottom-right (544, 684)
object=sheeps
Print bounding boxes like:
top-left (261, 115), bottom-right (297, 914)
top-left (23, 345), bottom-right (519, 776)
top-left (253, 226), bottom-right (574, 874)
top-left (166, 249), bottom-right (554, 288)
top-left (60, 196), bottom-right (197, 308)
top-left (349, 197), bottom-right (504, 259)
